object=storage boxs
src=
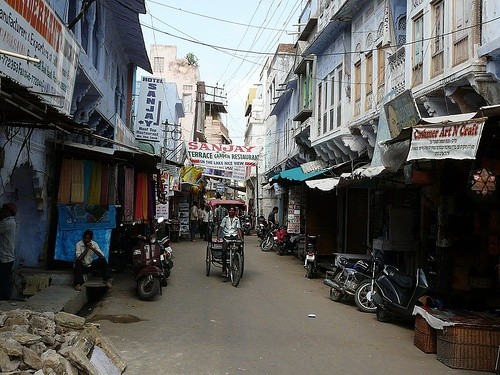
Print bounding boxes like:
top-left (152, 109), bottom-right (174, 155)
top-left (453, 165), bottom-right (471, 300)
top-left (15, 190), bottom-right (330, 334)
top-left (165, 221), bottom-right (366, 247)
top-left (413, 305), bottom-right (500, 374)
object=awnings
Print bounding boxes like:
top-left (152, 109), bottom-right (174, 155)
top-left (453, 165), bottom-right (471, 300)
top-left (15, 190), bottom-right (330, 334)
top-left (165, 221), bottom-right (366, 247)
top-left (0, 70), bottom-right (186, 171)
top-left (260, 105), bottom-right (500, 192)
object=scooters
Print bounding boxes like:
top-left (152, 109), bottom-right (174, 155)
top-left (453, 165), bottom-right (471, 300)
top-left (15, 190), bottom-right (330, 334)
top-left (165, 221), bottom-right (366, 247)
top-left (376, 253), bottom-right (437, 319)
top-left (301, 232), bottom-right (321, 279)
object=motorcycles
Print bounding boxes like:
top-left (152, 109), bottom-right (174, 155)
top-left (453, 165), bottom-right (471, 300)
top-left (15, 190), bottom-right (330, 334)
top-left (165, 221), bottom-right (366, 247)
top-left (129, 223), bottom-right (173, 301)
top-left (243, 210), bottom-right (290, 252)
top-left (324, 244), bottom-right (386, 312)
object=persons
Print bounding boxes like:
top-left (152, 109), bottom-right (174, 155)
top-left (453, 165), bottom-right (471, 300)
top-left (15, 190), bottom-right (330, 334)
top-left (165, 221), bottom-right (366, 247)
top-left (1, 203), bottom-right (17, 301)
top-left (190, 200), bottom-right (227, 241)
top-left (73, 230), bottom-right (113, 292)
top-left (216, 205), bottom-right (244, 277)
top-left (268, 206), bottom-right (279, 229)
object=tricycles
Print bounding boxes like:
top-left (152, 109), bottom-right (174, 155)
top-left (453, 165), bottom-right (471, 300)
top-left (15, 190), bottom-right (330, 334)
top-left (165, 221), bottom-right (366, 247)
top-left (206, 197), bottom-right (245, 286)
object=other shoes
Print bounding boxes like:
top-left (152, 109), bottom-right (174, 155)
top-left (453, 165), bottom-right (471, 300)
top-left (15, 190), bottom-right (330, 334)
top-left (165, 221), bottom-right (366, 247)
top-left (104, 280), bottom-right (112, 288)
top-left (222, 269), bottom-right (226, 276)
top-left (75, 284), bottom-right (81, 291)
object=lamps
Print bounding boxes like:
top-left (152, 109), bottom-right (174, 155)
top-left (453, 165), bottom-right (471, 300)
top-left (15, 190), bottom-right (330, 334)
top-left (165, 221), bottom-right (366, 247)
top-left (468, 163), bottom-right (498, 199)
